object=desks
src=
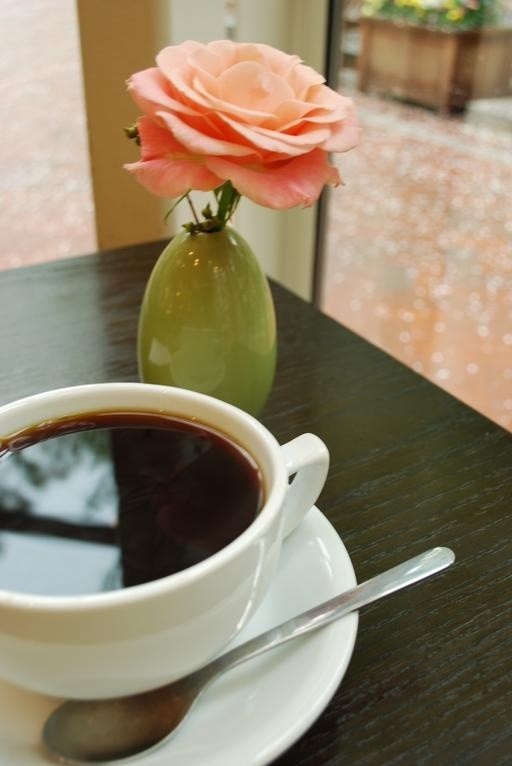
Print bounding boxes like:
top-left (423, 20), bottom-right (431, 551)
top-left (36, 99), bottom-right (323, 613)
top-left (1, 238), bottom-right (512, 766)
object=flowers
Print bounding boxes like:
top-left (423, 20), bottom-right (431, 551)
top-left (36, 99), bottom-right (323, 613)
top-left (126, 38), bottom-right (362, 232)
top-left (358, 0), bottom-right (512, 35)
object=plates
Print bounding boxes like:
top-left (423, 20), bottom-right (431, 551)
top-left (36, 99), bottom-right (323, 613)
top-left (1, 482), bottom-right (362, 766)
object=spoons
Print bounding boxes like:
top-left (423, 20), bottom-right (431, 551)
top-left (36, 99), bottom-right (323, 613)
top-left (39, 544), bottom-right (459, 766)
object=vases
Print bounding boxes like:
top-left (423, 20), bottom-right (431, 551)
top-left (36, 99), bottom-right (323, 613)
top-left (348, 20), bottom-right (512, 119)
top-left (136, 224), bottom-right (279, 419)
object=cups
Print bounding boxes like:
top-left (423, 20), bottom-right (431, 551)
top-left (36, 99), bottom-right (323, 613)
top-left (0, 382), bottom-right (331, 702)
